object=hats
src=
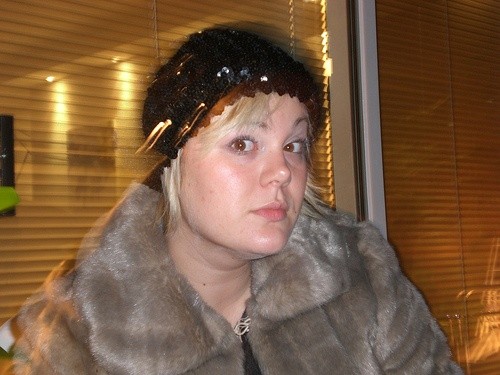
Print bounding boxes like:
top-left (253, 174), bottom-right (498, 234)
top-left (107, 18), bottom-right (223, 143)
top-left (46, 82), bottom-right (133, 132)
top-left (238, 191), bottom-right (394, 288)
top-left (141, 27), bottom-right (324, 160)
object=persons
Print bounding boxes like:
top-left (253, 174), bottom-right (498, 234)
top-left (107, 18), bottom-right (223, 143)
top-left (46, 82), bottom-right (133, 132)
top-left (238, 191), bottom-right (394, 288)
top-left (0, 20), bottom-right (464, 375)
